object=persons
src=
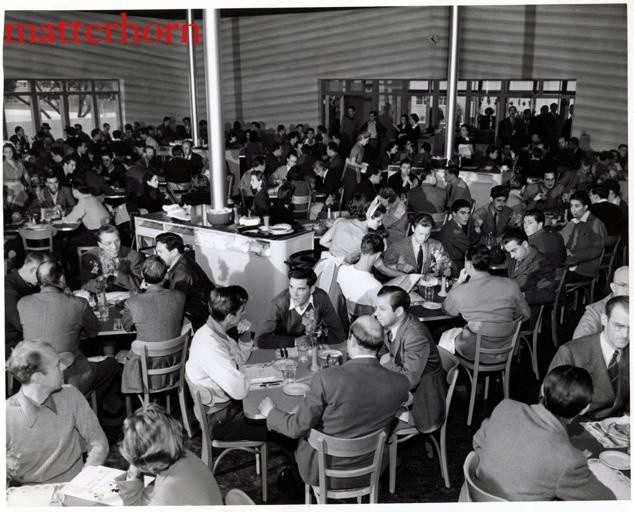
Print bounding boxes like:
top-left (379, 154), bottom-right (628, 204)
top-left (3, 103), bottom-right (629, 506)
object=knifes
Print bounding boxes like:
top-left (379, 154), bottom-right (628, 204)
top-left (279, 346), bottom-right (289, 359)
top-left (259, 379), bottom-right (281, 388)
top-left (592, 420), bottom-right (620, 447)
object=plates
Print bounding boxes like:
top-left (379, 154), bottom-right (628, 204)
top-left (601, 415), bottom-right (629, 441)
top-left (422, 302), bottom-right (442, 310)
top-left (240, 216), bottom-right (261, 226)
top-left (417, 276), bottom-right (439, 289)
top-left (598, 449), bottom-right (631, 470)
top-left (260, 225), bottom-right (295, 235)
top-left (280, 382), bottom-right (310, 397)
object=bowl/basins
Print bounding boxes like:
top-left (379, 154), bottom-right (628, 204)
top-left (206, 207), bottom-right (232, 226)
top-left (316, 348), bottom-right (343, 367)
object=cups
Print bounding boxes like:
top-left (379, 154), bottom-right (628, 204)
top-left (297, 339), bottom-right (309, 363)
top-left (282, 361), bottom-right (296, 386)
top-left (98, 307), bottom-right (109, 319)
top-left (424, 285), bottom-right (433, 302)
top-left (418, 282), bottom-right (425, 294)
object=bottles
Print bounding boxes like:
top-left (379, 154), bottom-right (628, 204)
top-left (547, 206), bottom-right (566, 230)
top-left (444, 268), bottom-right (453, 294)
top-left (483, 230), bottom-right (506, 247)
top-left (25, 206), bottom-right (67, 225)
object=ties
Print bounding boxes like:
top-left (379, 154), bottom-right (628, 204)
top-left (608, 350), bottom-right (618, 398)
top-left (417, 246), bottom-right (423, 273)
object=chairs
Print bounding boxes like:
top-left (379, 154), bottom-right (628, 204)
top-left (2, 143), bottom-right (630, 506)
